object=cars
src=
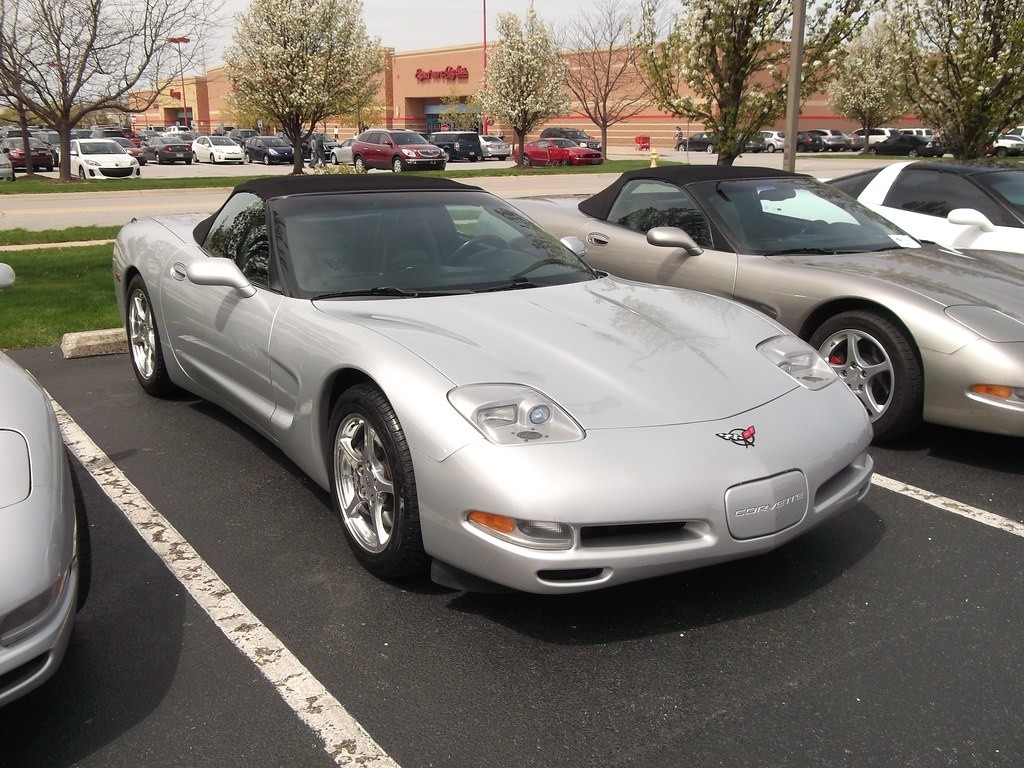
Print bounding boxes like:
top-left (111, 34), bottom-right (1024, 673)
top-left (0, 123), bottom-right (339, 166)
top-left (975, 125), bottom-right (1024, 158)
top-left (244, 136), bottom-right (296, 165)
top-left (191, 136), bottom-right (246, 165)
top-left (0, 260), bottom-right (82, 711)
top-left (674, 131), bottom-right (719, 154)
top-left (756, 159), bottom-right (1024, 255)
top-left (329, 138), bottom-right (357, 165)
top-left (0, 148), bottom-right (14, 181)
top-left (140, 137), bottom-right (194, 166)
top-left (57, 139), bottom-right (141, 181)
top-left (479, 135), bottom-right (511, 161)
top-left (732, 123), bottom-right (956, 158)
top-left (513, 136), bottom-right (604, 167)
top-left (0, 137), bottom-right (53, 172)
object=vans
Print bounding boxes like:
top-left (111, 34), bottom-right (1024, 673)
top-left (428, 131), bottom-right (482, 163)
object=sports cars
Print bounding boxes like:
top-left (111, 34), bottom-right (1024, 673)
top-left (111, 169), bottom-right (876, 598)
top-left (476, 163), bottom-right (1024, 443)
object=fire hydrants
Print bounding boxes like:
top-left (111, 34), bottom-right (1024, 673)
top-left (649, 148), bottom-right (661, 168)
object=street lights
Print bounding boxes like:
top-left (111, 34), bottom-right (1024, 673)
top-left (167, 36), bottom-right (191, 126)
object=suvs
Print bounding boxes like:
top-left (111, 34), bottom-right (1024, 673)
top-left (540, 126), bottom-right (602, 152)
top-left (351, 128), bottom-right (448, 173)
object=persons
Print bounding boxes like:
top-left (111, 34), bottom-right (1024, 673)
top-left (353, 132), bottom-right (358, 138)
top-left (673, 127), bottom-right (683, 149)
top-left (308, 133), bottom-right (326, 168)
top-left (334, 126), bottom-right (338, 138)
